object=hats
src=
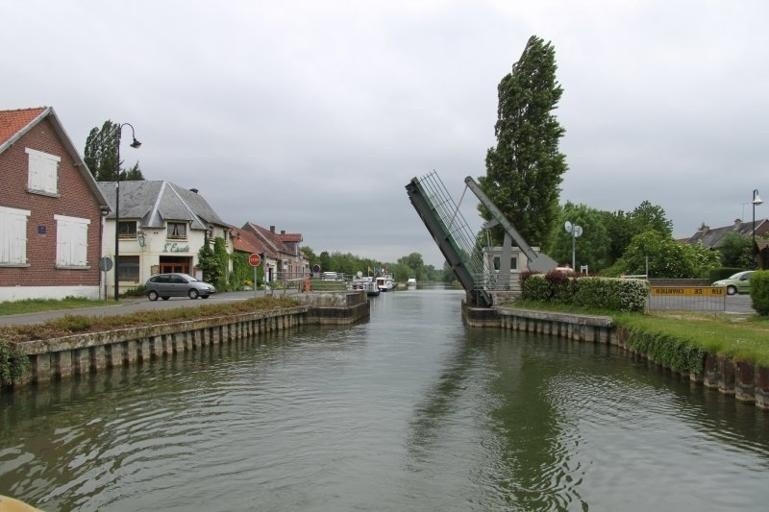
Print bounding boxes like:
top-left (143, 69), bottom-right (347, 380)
top-left (142, 272), bottom-right (216, 301)
top-left (710, 270), bottom-right (759, 295)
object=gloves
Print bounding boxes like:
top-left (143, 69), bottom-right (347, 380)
top-left (349, 266), bottom-right (398, 296)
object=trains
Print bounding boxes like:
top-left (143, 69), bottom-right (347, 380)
top-left (249, 254), bottom-right (261, 266)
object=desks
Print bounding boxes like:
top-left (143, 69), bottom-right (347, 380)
top-left (751, 189), bottom-right (763, 270)
top-left (114, 123), bottom-right (142, 300)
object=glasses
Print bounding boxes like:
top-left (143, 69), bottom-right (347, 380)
top-left (100, 205), bottom-right (110, 216)
top-left (137, 230), bottom-right (145, 248)
